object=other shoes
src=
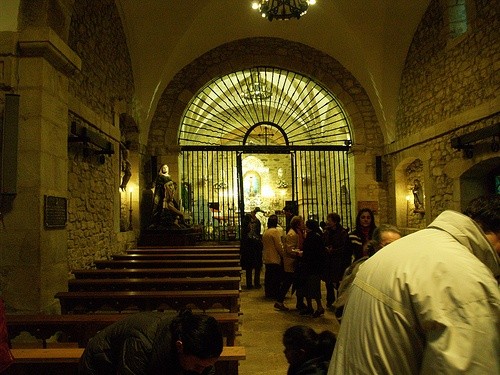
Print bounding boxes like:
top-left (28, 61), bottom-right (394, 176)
top-left (313, 310), bottom-right (324, 318)
top-left (247, 285), bottom-right (254, 289)
top-left (300, 308), bottom-right (314, 315)
top-left (274, 303), bottom-right (289, 311)
top-left (296, 303), bottom-right (306, 308)
top-left (264, 297), bottom-right (272, 301)
top-left (254, 285), bottom-right (261, 289)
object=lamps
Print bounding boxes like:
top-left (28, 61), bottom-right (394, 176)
top-left (241, 71), bottom-right (272, 100)
top-left (251, 0), bottom-right (316, 20)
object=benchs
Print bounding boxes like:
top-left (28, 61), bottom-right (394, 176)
top-left (0, 242), bottom-right (247, 375)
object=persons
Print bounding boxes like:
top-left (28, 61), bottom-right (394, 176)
top-left (120, 149), bottom-right (131, 191)
top-left (330, 193), bottom-right (500, 375)
top-left (74, 307), bottom-right (224, 375)
top-left (151, 164), bottom-right (179, 214)
top-left (281, 325), bottom-right (337, 375)
top-left (156, 180), bottom-right (192, 229)
top-left (239, 207), bottom-right (401, 322)
top-left (410, 178), bottom-right (425, 210)
